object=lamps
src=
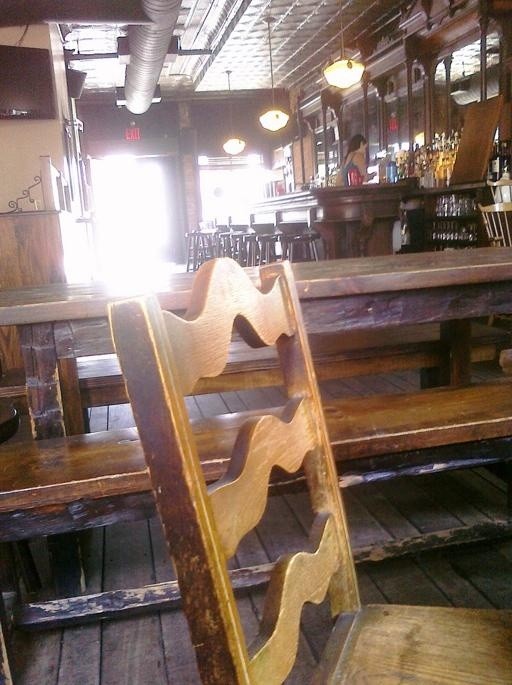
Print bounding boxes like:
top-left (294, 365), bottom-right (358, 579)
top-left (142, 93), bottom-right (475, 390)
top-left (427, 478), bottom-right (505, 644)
top-left (220, 0), bottom-right (366, 157)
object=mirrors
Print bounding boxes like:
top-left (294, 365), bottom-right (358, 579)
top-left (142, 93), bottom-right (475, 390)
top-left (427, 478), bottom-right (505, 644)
top-left (312, 28), bottom-right (502, 195)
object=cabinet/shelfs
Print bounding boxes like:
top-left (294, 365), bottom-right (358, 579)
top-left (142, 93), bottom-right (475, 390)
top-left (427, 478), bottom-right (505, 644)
top-left (1, 156), bottom-right (74, 381)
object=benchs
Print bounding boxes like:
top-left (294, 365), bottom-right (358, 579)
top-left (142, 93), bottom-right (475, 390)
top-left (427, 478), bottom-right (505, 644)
top-left (1, 380), bottom-right (512, 632)
top-left (1, 315), bottom-right (509, 436)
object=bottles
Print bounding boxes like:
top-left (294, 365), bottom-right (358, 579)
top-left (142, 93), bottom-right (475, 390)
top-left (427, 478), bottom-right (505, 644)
top-left (400, 129), bottom-right (461, 187)
top-left (384, 157), bottom-right (398, 185)
top-left (491, 136), bottom-right (510, 183)
top-left (433, 192), bottom-right (480, 242)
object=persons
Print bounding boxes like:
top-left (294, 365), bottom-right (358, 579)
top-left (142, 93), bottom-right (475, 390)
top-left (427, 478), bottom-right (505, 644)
top-left (340, 133), bottom-right (378, 186)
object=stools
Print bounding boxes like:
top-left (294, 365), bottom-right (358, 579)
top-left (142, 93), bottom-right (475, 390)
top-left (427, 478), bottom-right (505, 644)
top-left (183, 223), bottom-right (320, 274)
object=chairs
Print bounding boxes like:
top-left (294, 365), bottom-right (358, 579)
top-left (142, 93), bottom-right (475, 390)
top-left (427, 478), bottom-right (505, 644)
top-left (477, 178), bottom-right (511, 247)
top-left (108, 255), bottom-right (510, 684)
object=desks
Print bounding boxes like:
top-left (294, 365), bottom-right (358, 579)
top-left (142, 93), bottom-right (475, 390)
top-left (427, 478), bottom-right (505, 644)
top-left (0, 248), bottom-right (510, 586)
